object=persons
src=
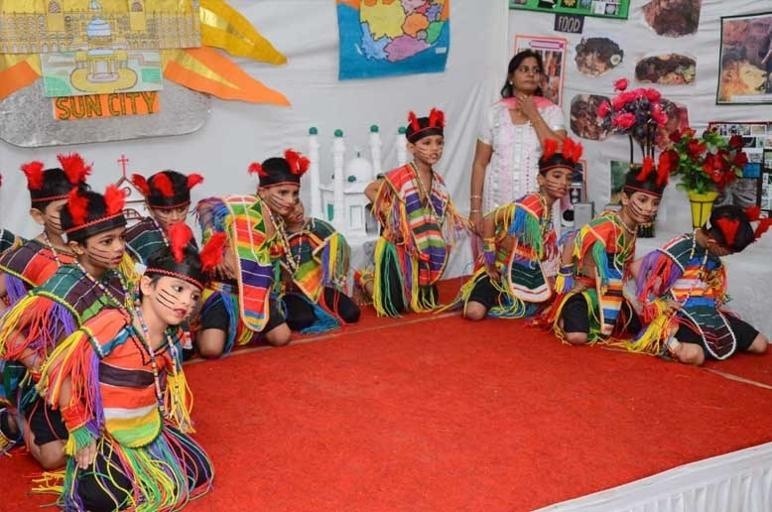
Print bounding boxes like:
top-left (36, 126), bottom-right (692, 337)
top-left (0, 167), bottom-right (213, 512)
top-left (281, 199), bottom-right (361, 331)
top-left (556, 164), bottom-right (667, 346)
top-left (359, 110), bottom-right (462, 317)
top-left (634, 205), bottom-right (768, 366)
top-left (197, 158), bottom-right (298, 358)
top-left (469, 49), bottom-right (567, 238)
top-left (464, 151), bottom-right (575, 321)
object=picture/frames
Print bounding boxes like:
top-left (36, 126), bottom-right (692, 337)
top-left (513, 35), bottom-right (568, 108)
top-left (715, 12), bottom-right (771, 105)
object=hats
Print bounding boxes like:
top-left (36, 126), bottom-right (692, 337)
top-left (539, 137), bottom-right (583, 175)
top-left (144, 221), bottom-right (228, 293)
top-left (19, 152), bottom-right (94, 202)
top-left (60, 185), bottom-right (128, 242)
top-left (703, 202), bottom-right (768, 254)
top-left (405, 108), bottom-right (446, 145)
top-left (131, 172), bottom-right (204, 211)
top-left (244, 149), bottom-right (310, 189)
top-left (623, 154), bottom-right (669, 199)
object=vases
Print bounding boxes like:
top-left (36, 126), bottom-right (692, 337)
top-left (687, 193), bottom-right (717, 229)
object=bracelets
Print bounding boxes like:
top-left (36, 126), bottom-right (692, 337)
top-left (531, 117), bottom-right (542, 126)
top-left (469, 195), bottom-right (483, 200)
top-left (471, 210), bottom-right (480, 213)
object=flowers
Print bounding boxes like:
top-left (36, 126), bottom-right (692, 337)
top-left (664, 129), bottom-right (747, 193)
top-left (597, 78), bottom-right (670, 166)
